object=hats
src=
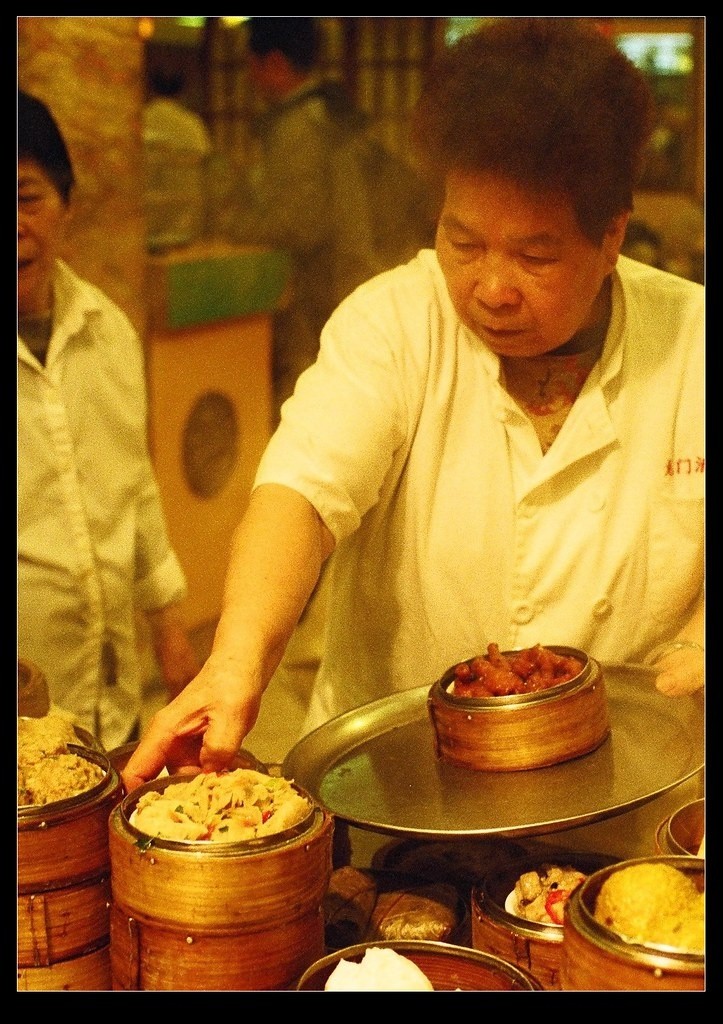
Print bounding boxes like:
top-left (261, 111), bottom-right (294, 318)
top-left (250, 16), bottom-right (318, 52)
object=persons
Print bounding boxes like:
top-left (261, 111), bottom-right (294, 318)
top-left (100, 18), bottom-right (707, 875)
top-left (19, 93), bottom-right (192, 752)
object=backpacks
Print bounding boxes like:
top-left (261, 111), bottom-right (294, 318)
top-left (270, 77), bottom-right (428, 319)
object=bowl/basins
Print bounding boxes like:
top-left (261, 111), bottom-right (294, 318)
top-left (18, 645), bottom-right (707, 992)
